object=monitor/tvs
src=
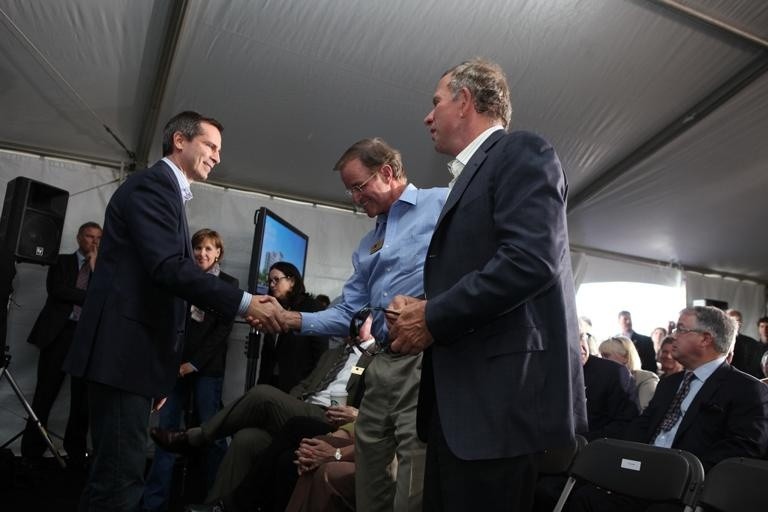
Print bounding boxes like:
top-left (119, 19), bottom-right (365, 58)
top-left (248, 205), bottom-right (310, 296)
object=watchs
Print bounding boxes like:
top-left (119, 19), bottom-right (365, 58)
top-left (333, 446), bottom-right (350, 460)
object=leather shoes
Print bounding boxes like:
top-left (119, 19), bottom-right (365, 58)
top-left (188, 497), bottom-right (224, 512)
top-left (150, 427), bottom-right (200, 456)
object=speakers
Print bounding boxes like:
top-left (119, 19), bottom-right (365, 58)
top-left (0, 176), bottom-right (69, 265)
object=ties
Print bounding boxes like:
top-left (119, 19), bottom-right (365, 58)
top-left (301, 347), bottom-right (354, 401)
top-left (649, 372), bottom-right (694, 444)
top-left (73, 260), bottom-right (90, 321)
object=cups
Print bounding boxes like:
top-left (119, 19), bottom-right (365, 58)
top-left (329, 390), bottom-right (348, 407)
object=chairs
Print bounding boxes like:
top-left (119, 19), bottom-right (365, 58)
top-left (693, 456), bottom-right (768, 510)
top-left (569, 434), bottom-right (589, 479)
top-left (551, 438), bottom-right (707, 511)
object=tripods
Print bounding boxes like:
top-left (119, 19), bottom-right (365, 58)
top-left (0, 266), bottom-right (70, 470)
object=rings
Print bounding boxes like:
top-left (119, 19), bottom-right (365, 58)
top-left (338, 417), bottom-right (342, 423)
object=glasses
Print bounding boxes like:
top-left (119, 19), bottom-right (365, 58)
top-left (350, 307), bottom-right (400, 357)
top-left (670, 325), bottom-right (701, 336)
top-left (266, 276), bottom-right (290, 286)
top-left (344, 171), bottom-right (378, 198)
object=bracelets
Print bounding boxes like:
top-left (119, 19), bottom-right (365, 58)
top-left (327, 431), bottom-right (333, 438)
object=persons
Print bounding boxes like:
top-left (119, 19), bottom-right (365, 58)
top-left (68, 112), bottom-right (285, 512)
top-left (253, 261), bottom-right (326, 386)
top-left (757, 316), bottom-right (768, 351)
top-left (618, 311), bottom-right (658, 374)
top-left (284, 423), bottom-right (356, 511)
top-left (244, 135), bottom-right (448, 511)
top-left (141, 228), bottom-right (239, 512)
top-left (383, 55), bottom-right (588, 511)
top-left (659, 337), bottom-right (684, 381)
top-left (649, 328), bottom-right (667, 370)
top-left (149, 306), bottom-right (376, 510)
top-left (726, 308), bottom-right (766, 380)
top-left (19, 223), bottom-right (102, 464)
top-left (612, 305), bottom-right (767, 480)
top-left (599, 335), bottom-right (660, 415)
top-left (576, 314), bottom-right (640, 439)
top-left (760, 351), bottom-right (768, 382)
